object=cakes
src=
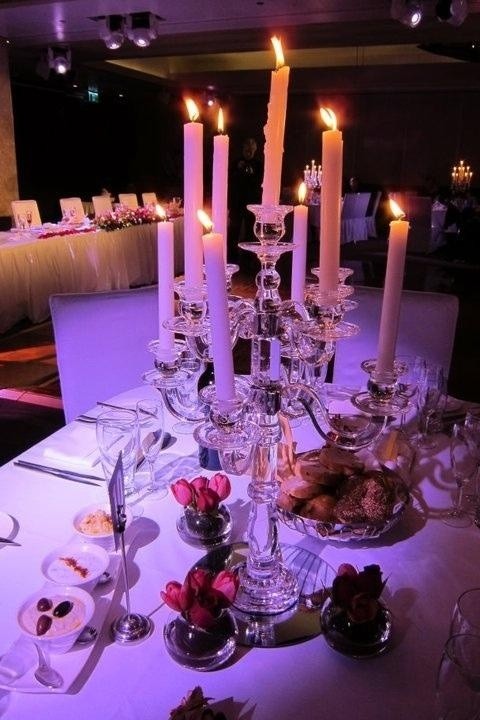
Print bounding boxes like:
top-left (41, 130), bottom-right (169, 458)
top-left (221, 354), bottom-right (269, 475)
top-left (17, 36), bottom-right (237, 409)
top-left (79, 508), bottom-right (115, 536)
top-left (22, 595), bottom-right (87, 637)
top-left (47, 551), bottom-right (105, 583)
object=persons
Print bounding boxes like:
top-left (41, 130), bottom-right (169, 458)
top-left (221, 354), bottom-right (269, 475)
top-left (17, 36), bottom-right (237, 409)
top-left (228, 137), bottom-right (263, 253)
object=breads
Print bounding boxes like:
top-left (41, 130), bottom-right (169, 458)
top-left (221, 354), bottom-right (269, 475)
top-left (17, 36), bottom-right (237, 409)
top-left (274, 446), bottom-right (410, 527)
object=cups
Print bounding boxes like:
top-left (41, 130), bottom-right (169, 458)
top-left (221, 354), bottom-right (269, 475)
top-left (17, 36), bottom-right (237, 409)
top-left (447, 589), bottom-right (479, 674)
top-left (435, 634), bottom-right (480, 719)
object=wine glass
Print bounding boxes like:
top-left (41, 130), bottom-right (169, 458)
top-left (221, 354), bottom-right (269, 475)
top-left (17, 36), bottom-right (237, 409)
top-left (76, 393), bottom-right (169, 520)
top-left (395, 351), bottom-right (445, 456)
top-left (14, 205), bottom-right (94, 240)
top-left (439, 409), bottom-right (480, 530)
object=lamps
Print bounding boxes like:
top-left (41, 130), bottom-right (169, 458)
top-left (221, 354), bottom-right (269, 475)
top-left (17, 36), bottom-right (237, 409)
top-left (45, 46), bottom-right (72, 75)
top-left (95, 11), bottom-right (159, 50)
top-left (389, 0), bottom-right (471, 29)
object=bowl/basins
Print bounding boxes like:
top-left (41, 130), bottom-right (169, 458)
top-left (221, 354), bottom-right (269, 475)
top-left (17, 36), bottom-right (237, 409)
top-left (14, 501), bottom-right (133, 655)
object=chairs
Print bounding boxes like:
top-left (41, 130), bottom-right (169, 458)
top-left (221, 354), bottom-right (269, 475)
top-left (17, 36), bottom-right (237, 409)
top-left (92, 195), bottom-right (114, 220)
top-left (47, 283), bottom-right (159, 424)
top-left (9, 199), bottom-right (42, 230)
top-left (58, 197), bottom-right (85, 221)
top-left (333, 283), bottom-right (459, 402)
top-left (142, 193), bottom-right (158, 210)
top-left (118, 194), bottom-right (138, 213)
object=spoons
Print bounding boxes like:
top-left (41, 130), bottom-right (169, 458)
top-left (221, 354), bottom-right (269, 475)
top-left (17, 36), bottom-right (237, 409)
top-left (77, 625), bottom-right (97, 646)
top-left (99, 573), bottom-right (111, 586)
top-left (31, 644), bottom-right (65, 691)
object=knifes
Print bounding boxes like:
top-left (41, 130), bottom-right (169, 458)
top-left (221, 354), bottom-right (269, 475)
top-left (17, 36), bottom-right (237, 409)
top-left (14, 459), bottom-right (105, 488)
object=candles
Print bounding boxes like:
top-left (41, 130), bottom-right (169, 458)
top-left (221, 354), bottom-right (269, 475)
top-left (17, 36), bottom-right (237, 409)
top-left (258, 38), bottom-right (289, 207)
top-left (182, 98), bottom-right (229, 295)
top-left (154, 206), bottom-right (176, 350)
top-left (290, 183), bottom-right (309, 305)
top-left (451, 159), bottom-right (472, 182)
top-left (372, 199), bottom-right (408, 370)
top-left (196, 208), bottom-right (236, 401)
top-left (316, 106), bottom-right (344, 291)
